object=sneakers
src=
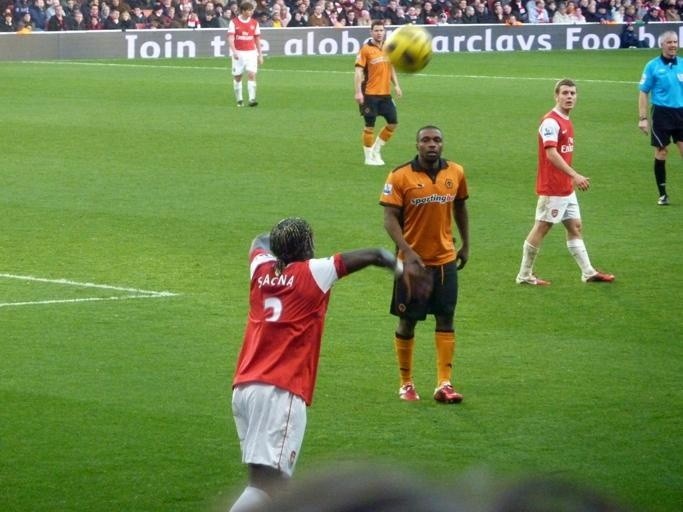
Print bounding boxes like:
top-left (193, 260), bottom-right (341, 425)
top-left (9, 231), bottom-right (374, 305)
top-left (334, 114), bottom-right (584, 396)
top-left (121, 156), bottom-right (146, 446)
top-left (582, 272), bottom-right (615, 282)
top-left (399, 384), bottom-right (420, 400)
top-left (658, 195), bottom-right (669, 205)
top-left (516, 274), bottom-right (551, 285)
top-left (433, 385), bottom-right (463, 401)
top-left (365, 148), bottom-right (385, 166)
top-left (236, 98), bottom-right (258, 107)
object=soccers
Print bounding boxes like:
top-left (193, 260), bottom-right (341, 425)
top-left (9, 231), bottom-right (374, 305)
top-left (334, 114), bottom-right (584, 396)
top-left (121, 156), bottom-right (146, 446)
top-left (384, 24), bottom-right (432, 73)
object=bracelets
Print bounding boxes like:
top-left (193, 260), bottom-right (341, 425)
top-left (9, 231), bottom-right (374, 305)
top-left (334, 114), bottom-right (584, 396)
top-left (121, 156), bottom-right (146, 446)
top-left (639, 115), bottom-right (646, 121)
top-left (393, 258), bottom-right (404, 278)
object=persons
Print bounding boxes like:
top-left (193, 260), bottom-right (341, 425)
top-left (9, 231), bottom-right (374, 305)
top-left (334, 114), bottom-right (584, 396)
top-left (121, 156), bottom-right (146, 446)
top-left (224, 3), bottom-right (264, 109)
top-left (513, 79), bottom-right (616, 287)
top-left (637, 29), bottom-right (683, 206)
top-left (619, 24), bottom-right (650, 49)
top-left (350, 21), bottom-right (404, 166)
top-left (227, 213), bottom-right (434, 512)
top-left (375, 124), bottom-right (472, 405)
top-left (0, 1), bottom-right (682, 32)
